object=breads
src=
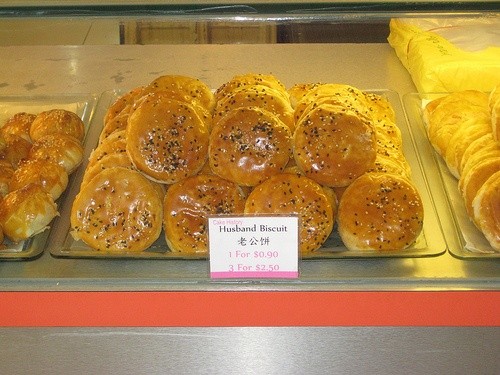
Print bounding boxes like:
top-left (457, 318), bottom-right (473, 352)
top-left (424, 88), bottom-right (500, 251)
top-left (0, 109), bottom-right (85, 243)
top-left (68, 75), bottom-right (424, 251)
